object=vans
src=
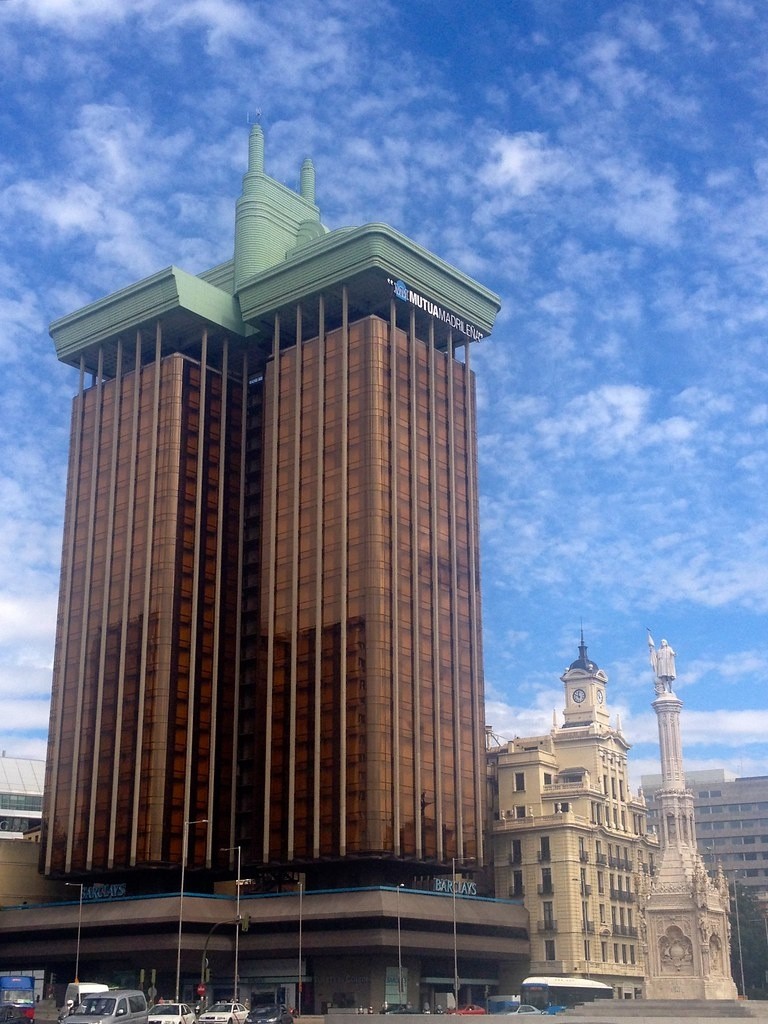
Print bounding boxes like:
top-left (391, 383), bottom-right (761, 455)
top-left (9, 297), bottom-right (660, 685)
top-left (64, 982), bottom-right (109, 1014)
top-left (59, 990), bottom-right (149, 1024)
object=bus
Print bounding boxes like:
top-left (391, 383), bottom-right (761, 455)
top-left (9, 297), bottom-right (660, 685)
top-left (486, 995), bottom-right (520, 1014)
top-left (520, 977), bottom-right (614, 1011)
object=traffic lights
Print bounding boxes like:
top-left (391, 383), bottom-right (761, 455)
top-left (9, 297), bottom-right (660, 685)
top-left (205, 968), bottom-right (212, 985)
top-left (243, 911), bottom-right (252, 933)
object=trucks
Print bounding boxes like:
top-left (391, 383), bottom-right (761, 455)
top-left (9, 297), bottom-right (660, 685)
top-left (0, 975), bottom-right (41, 1024)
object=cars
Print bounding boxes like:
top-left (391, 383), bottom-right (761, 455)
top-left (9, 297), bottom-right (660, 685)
top-left (492, 1004), bottom-right (542, 1015)
top-left (540, 1005), bottom-right (569, 1015)
top-left (378, 1004), bottom-right (420, 1016)
top-left (145, 1003), bottom-right (198, 1024)
top-left (197, 1002), bottom-right (250, 1024)
top-left (447, 1004), bottom-right (486, 1015)
top-left (243, 1003), bottom-right (294, 1024)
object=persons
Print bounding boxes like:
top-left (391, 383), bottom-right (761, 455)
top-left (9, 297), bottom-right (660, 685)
top-left (158, 996), bottom-right (163, 1004)
top-left (67, 999), bottom-right (75, 1015)
top-left (245, 998), bottom-right (250, 1010)
top-left (423, 999), bottom-right (430, 1011)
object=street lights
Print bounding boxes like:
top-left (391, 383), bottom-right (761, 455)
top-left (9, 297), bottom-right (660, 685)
top-left (297, 880), bottom-right (304, 1019)
top-left (175, 819), bottom-right (209, 1002)
top-left (65, 883), bottom-right (83, 984)
top-left (451, 856), bottom-right (476, 1011)
top-left (397, 884), bottom-right (405, 1008)
top-left (220, 846), bottom-right (241, 1003)
